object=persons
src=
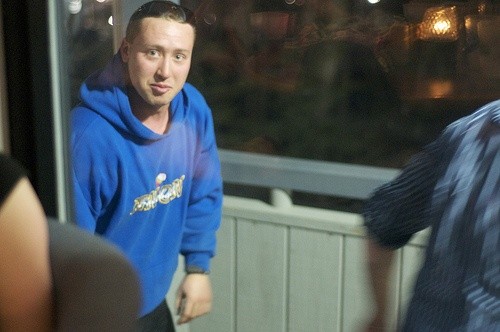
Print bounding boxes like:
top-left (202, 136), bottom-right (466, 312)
top-left (67, 0), bottom-right (223, 332)
top-left (362, 99), bottom-right (500, 332)
